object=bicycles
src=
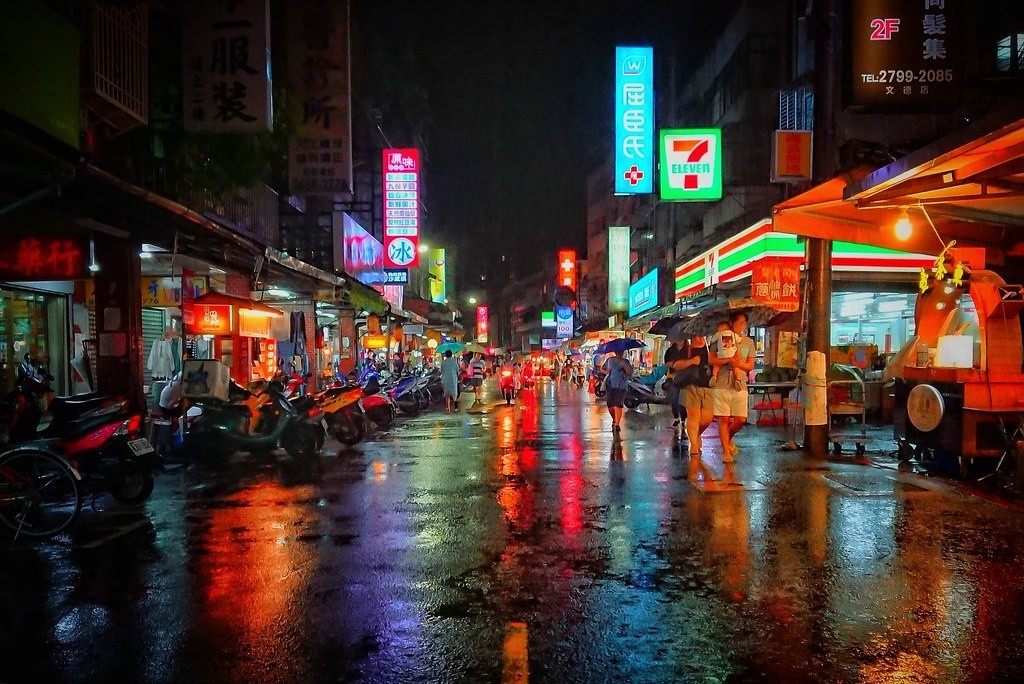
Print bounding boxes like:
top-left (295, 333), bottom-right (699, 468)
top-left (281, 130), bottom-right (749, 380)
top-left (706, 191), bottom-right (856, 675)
top-left (1, 438), bottom-right (83, 550)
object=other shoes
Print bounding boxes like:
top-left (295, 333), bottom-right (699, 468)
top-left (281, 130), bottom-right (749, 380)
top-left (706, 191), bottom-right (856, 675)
top-left (681, 434), bottom-right (688, 439)
top-left (672, 419), bottom-right (679, 427)
top-left (734, 379), bottom-right (742, 392)
top-left (708, 376), bottom-right (717, 388)
top-left (445, 407), bottom-right (449, 411)
top-left (682, 423), bottom-right (685, 428)
top-left (697, 437), bottom-right (702, 449)
top-left (673, 435), bottom-right (679, 439)
top-left (612, 422), bottom-right (621, 438)
top-left (690, 446), bottom-right (698, 454)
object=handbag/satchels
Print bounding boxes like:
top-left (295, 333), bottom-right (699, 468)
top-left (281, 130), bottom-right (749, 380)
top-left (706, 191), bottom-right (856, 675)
top-left (600, 359), bottom-right (611, 391)
top-left (673, 345), bottom-right (705, 387)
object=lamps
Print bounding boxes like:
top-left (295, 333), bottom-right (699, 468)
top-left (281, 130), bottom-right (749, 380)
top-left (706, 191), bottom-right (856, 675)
top-left (892, 206), bottom-right (915, 243)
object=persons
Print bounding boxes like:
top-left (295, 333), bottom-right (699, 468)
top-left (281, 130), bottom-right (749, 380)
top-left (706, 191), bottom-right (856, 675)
top-left (322, 362), bottom-right (334, 386)
top-left (393, 353), bottom-right (403, 386)
top-left (707, 311), bottom-right (756, 463)
top-left (82, 350), bottom-right (93, 390)
top-left (601, 350), bottom-right (632, 432)
top-left (422, 358), bottom-right (429, 374)
top-left (708, 321), bottom-right (745, 391)
top-left (440, 350), bottom-right (460, 412)
top-left (673, 333), bottom-right (713, 454)
top-left (564, 354), bottom-right (574, 384)
top-left (593, 350), bottom-right (603, 391)
top-left (664, 340), bottom-right (689, 439)
top-left (158, 371), bottom-right (193, 420)
top-left (492, 353), bottom-right (545, 398)
top-left (362, 349), bottom-right (376, 371)
top-left (553, 349), bottom-right (564, 388)
top-left (470, 350), bottom-right (486, 403)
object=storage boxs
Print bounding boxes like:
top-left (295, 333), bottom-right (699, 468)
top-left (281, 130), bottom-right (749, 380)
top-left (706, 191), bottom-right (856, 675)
top-left (178, 358), bottom-right (230, 402)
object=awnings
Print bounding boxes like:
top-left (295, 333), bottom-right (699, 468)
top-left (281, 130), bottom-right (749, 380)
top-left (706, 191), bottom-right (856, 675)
top-left (772, 112), bottom-right (1023, 260)
top-left (628, 301), bottom-right (678, 327)
top-left (88, 162), bottom-right (431, 324)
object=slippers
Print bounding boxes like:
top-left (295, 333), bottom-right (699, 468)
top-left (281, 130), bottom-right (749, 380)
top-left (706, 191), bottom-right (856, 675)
top-left (729, 443), bottom-right (738, 457)
top-left (723, 454), bottom-right (734, 463)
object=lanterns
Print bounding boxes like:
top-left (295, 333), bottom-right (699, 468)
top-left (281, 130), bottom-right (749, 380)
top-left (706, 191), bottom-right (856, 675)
top-left (392, 325), bottom-right (405, 341)
top-left (366, 314), bottom-right (380, 334)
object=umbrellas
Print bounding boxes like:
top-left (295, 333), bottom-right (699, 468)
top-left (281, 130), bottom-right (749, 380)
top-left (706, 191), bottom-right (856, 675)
top-left (435, 342), bottom-right (466, 359)
top-left (597, 339), bottom-right (647, 369)
top-left (562, 348), bottom-right (580, 356)
top-left (665, 317), bottom-right (710, 354)
top-left (647, 317), bottom-right (684, 335)
top-left (684, 300), bottom-right (780, 351)
top-left (465, 344), bottom-right (486, 359)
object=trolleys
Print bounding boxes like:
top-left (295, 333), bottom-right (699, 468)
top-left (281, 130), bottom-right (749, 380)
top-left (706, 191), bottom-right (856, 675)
top-left (825, 379), bottom-right (873, 458)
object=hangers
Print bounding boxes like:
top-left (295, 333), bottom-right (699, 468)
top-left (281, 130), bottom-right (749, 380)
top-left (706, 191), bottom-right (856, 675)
top-left (292, 301), bottom-right (302, 312)
top-left (156, 332), bottom-right (181, 341)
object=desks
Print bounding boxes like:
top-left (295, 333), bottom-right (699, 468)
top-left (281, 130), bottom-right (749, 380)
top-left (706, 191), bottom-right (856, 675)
top-left (747, 383), bottom-right (797, 427)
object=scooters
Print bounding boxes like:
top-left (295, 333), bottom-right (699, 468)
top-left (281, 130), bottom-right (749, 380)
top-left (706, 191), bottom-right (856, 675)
top-left (622, 375), bottom-right (671, 411)
top-left (150, 352), bottom-right (495, 460)
top-left (572, 364), bottom-right (585, 388)
top-left (591, 371), bottom-right (609, 397)
top-left (520, 367), bottom-right (536, 391)
top-left (0, 353), bottom-right (156, 505)
top-left (496, 362), bottom-right (521, 406)
